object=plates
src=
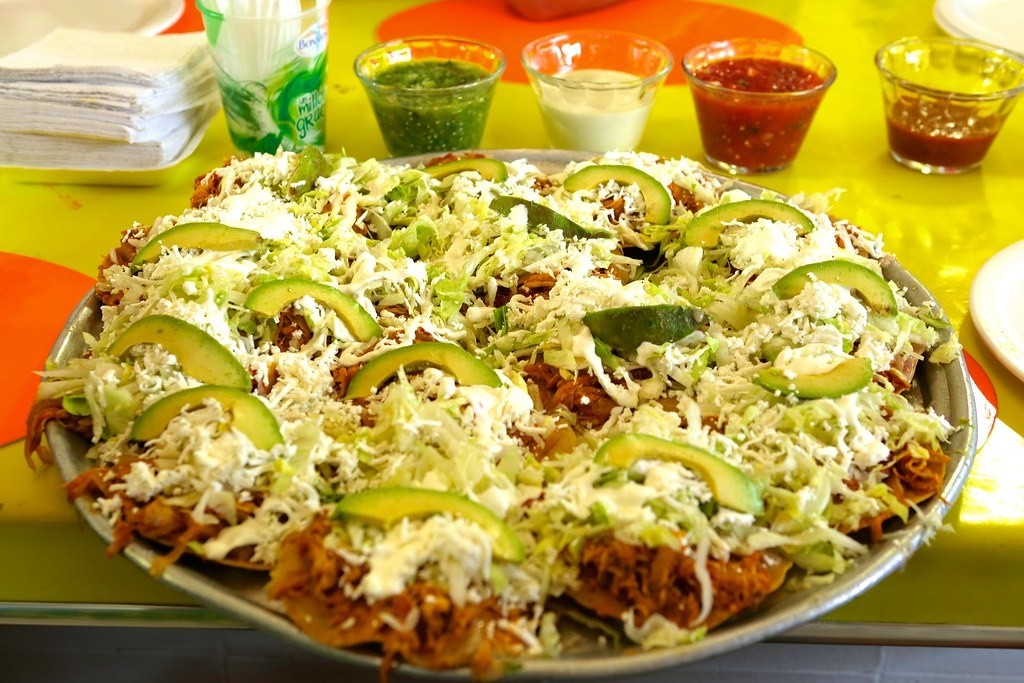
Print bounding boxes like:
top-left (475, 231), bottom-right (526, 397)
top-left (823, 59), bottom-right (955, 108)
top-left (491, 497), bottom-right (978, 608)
top-left (40, 149), bottom-right (977, 678)
top-left (935, 0), bottom-right (1024, 57)
top-left (968, 239), bottom-right (1024, 383)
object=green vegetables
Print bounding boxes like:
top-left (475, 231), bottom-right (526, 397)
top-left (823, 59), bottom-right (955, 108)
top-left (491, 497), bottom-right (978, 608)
top-left (371, 60), bottom-right (490, 87)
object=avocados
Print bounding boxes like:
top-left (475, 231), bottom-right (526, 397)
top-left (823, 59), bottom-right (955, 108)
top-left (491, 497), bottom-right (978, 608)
top-left (107, 157), bottom-right (897, 565)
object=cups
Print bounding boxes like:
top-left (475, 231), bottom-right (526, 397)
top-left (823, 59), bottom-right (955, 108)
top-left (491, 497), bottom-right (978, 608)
top-left (681, 38), bottom-right (836, 174)
top-left (875, 35), bottom-right (1024, 174)
top-left (194, 0), bottom-right (332, 157)
top-left (521, 30), bottom-right (676, 151)
top-left (354, 35), bottom-right (507, 158)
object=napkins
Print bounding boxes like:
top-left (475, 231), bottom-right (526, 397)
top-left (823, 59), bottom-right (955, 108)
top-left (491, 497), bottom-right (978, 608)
top-left (0, 27), bottom-right (213, 170)
